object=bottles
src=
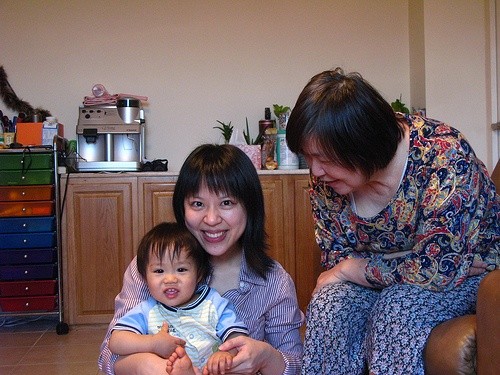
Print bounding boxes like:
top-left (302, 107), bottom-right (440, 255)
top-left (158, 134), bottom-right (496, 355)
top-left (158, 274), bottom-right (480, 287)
top-left (17, 113), bottom-right (25, 123)
top-left (92, 84), bottom-right (111, 97)
top-left (29, 110), bottom-right (42, 123)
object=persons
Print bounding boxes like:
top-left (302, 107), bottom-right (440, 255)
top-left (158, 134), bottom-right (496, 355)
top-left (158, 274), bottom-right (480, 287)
top-left (108, 222), bottom-right (249, 375)
top-left (286, 68), bottom-right (500, 375)
top-left (98, 143), bottom-right (303, 375)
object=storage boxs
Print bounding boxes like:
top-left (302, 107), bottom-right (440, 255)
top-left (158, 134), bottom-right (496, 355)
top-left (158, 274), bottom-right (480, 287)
top-left (0, 150), bottom-right (60, 312)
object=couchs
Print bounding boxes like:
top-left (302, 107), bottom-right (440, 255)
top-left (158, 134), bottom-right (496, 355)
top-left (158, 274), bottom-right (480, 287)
top-left (421, 156), bottom-right (500, 375)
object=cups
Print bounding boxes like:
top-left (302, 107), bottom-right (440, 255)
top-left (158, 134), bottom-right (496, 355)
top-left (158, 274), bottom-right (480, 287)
top-left (3, 132), bottom-right (15, 149)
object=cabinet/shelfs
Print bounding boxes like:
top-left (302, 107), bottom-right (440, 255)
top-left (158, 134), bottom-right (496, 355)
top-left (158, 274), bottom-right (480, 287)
top-left (0, 134), bottom-right (71, 335)
top-left (60, 173), bottom-right (325, 331)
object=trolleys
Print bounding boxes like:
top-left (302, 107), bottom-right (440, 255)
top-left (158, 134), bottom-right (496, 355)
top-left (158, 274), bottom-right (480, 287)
top-left (0, 135), bottom-right (70, 335)
